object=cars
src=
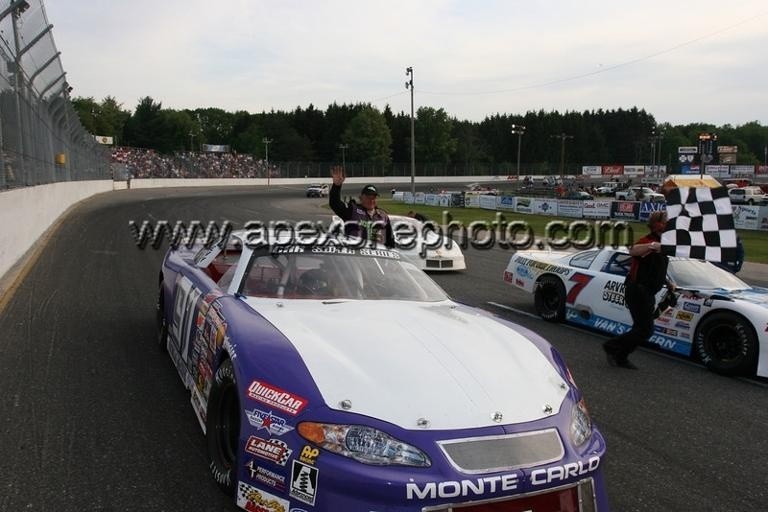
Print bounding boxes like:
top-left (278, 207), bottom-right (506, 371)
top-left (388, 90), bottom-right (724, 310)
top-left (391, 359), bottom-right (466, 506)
top-left (728, 188), bottom-right (768, 205)
top-left (560, 181), bottom-right (665, 203)
top-left (156, 215), bottom-right (768, 511)
top-left (306, 184), bottom-right (330, 198)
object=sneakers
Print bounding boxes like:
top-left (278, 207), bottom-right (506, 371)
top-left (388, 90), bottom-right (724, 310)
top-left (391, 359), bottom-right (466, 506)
top-left (602, 342), bottom-right (636, 369)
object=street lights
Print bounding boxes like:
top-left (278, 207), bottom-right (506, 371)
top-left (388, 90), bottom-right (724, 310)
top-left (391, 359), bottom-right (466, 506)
top-left (404, 67), bottom-right (415, 193)
top-left (511, 124), bottom-right (526, 180)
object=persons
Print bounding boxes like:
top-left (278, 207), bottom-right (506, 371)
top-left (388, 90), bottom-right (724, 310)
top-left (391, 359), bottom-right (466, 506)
top-left (520, 175), bottom-right (657, 203)
top-left (600, 211), bottom-right (671, 370)
top-left (406, 210), bottom-right (435, 231)
top-left (108, 145), bottom-right (280, 179)
top-left (328, 165), bottom-right (401, 238)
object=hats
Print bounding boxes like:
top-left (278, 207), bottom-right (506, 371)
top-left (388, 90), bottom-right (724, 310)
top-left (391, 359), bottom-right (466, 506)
top-left (361, 185), bottom-right (380, 198)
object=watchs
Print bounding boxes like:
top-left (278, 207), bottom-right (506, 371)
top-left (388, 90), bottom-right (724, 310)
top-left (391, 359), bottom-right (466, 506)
top-left (648, 241), bottom-right (656, 251)
